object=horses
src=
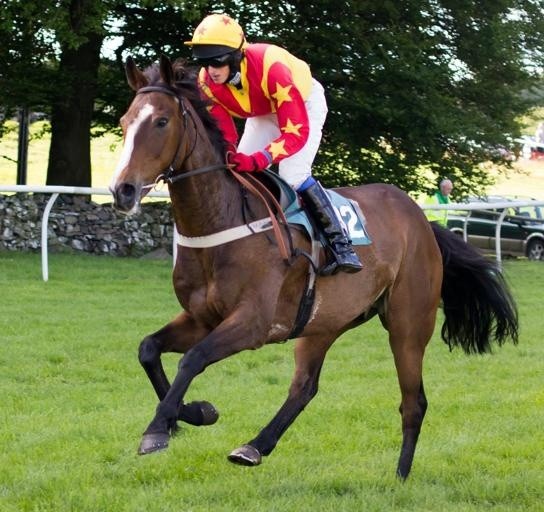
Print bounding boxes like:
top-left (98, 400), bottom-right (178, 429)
top-left (107, 51), bottom-right (520, 481)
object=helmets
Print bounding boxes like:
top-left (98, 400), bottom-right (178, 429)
top-left (184, 13), bottom-right (247, 53)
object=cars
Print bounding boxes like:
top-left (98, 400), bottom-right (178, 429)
top-left (496, 134), bottom-right (544, 162)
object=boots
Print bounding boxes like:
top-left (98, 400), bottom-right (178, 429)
top-left (300, 180), bottom-right (364, 277)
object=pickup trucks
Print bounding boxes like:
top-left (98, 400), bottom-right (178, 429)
top-left (446, 194), bottom-right (544, 262)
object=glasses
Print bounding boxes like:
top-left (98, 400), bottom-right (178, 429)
top-left (191, 35), bottom-right (245, 68)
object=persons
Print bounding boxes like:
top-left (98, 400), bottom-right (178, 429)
top-left (433, 179), bottom-right (454, 204)
top-left (183, 13), bottom-right (364, 276)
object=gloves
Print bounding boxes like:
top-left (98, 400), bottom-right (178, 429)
top-left (224, 151), bottom-right (258, 172)
